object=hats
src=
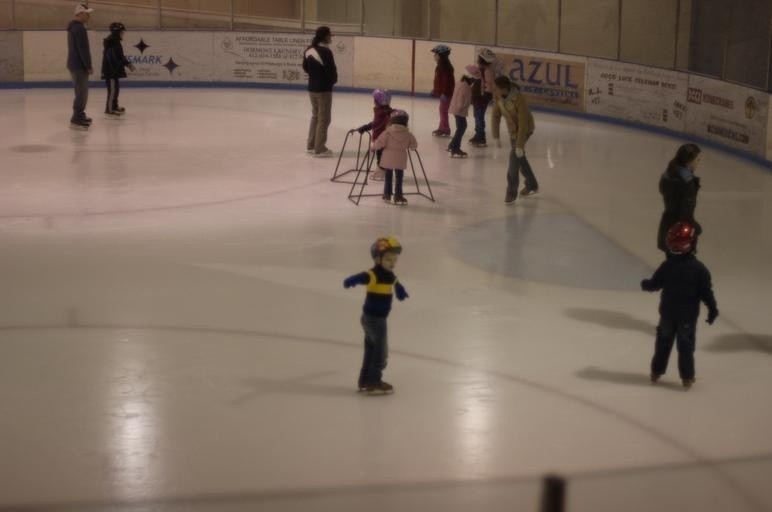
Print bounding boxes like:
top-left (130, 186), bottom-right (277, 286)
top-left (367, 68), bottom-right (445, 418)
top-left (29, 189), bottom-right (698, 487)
top-left (75, 4), bottom-right (94, 16)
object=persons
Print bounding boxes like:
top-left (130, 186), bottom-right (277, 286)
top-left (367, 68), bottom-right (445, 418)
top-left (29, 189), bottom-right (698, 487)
top-left (343, 236), bottom-right (410, 395)
top-left (302, 26), bottom-right (338, 156)
top-left (357, 88), bottom-right (393, 171)
top-left (640, 220), bottom-right (719, 390)
top-left (657, 144), bottom-right (703, 260)
top-left (490, 75), bottom-right (539, 204)
top-left (371, 109), bottom-right (418, 203)
top-left (101, 23), bottom-right (136, 117)
top-left (430, 44), bottom-right (496, 158)
top-left (66, 2), bottom-right (95, 128)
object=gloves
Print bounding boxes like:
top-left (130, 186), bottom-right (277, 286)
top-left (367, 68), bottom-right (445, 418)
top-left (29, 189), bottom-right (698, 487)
top-left (494, 139), bottom-right (501, 147)
top-left (128, 63), bottom-right (135, 72)
top-left (344, 279), bottom-right (354, 289)
top-left (704, 309), bottom-right (718, 325)
top-left (516, 147), bottom-right (522, 157)
top-left (359, 126), bottom-right (368, 132)
top-left (398, 292), bottom-right (409, 302)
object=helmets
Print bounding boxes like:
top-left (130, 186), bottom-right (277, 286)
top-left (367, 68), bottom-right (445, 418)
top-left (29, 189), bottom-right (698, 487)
top-left (390, 110), bottom-right (410, 121)
top-left (667, 223), bottom-right (697, 253)
top-left (371, 236), bottom-right (401, 259)
top-left (431, 46), bottom-right (495, 81)
top-left (110, 23), bottom-right (125, 34)
top-left (373, 88), bottom-right (390, 105)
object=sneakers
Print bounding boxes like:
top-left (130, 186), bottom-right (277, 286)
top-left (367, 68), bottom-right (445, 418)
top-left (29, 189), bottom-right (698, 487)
top-left (105, 107), bottom-right (124, 116)
top-left (370, 169), bottom-right (385, 177)
top-left (432, 130), bottom-right (486, 154)
top-left (382, 195), bottom-right (391, 201)
top-left (394, 195), bottom-right (407, 201)
top-left (71, 115), bottom-right (92, 127)
top-left (684, 376), bottom-right (694, 386)
top-left (358, 381), bottom-right (392, 390)
top-left (505, 194), bottom-right (514, 201)
top-left (521, 186), bottom-right (531, 195)
top-left (307, 147), bottom-right (332, 155)
top-left (650, 373), bottom-right (661, 378)
